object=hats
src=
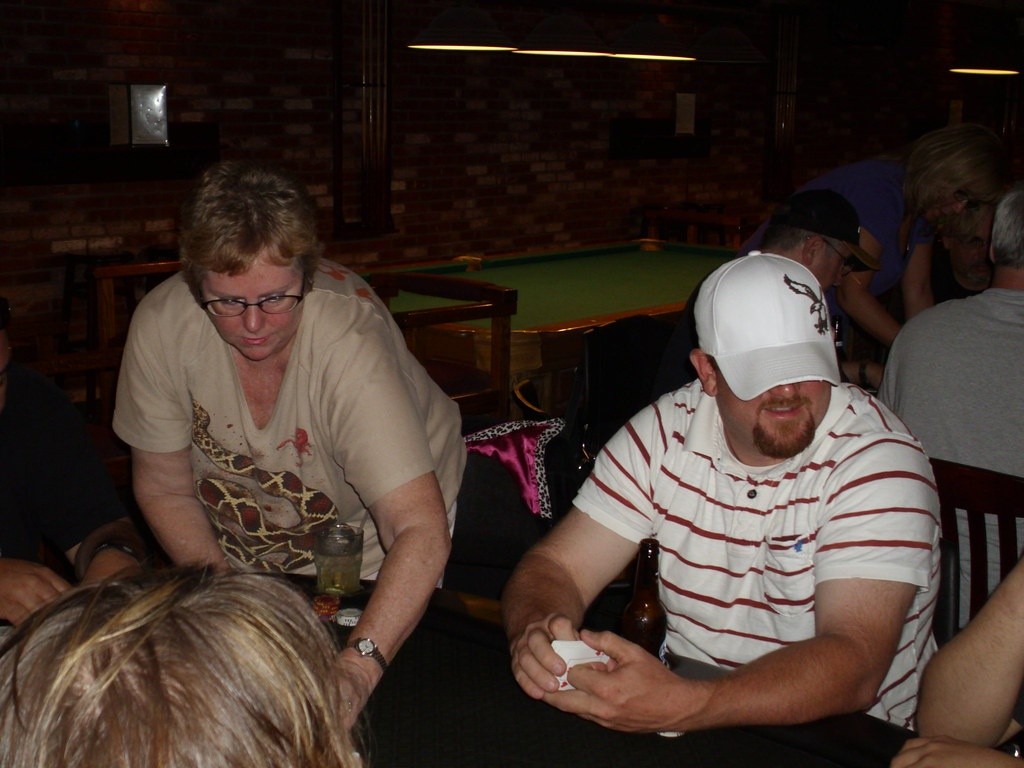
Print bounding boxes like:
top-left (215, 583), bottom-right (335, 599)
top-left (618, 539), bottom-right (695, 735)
top-left (694, 250), bottom-right (840, 401)
top-left (770, 189), bottom-right (879, 271)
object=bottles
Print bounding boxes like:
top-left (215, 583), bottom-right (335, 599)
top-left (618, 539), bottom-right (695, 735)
top-left (619, 538), bottom-right (670, 672)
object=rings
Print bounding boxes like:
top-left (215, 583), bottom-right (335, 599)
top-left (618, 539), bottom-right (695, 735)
top-left (346, 700), bottom-right (352, 712)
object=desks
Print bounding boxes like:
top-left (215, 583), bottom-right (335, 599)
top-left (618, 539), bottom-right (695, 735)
top-left (352, 237), bottom-right (738, 422)
top-left (323, 588), bottom-right (917, 768)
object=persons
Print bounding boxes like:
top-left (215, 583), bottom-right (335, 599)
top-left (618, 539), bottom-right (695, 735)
top-left (0, 562), bottom-right (373, 768)
top-left (890, 547), bottom-right (1024, 768)
top-left (502, 249), bottom-right (942, 731)
top-left (0, 295), bottom-right (146, 630)
top-left (739, 123), bottom-right (1024, 629)
top-left (109, 157), bottom-right (467, 730)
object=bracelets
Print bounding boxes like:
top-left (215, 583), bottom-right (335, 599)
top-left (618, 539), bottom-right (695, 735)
top-left (858, 359), bottom-right (867, 384)
top-left (92, 543), bottom-right (142, 564)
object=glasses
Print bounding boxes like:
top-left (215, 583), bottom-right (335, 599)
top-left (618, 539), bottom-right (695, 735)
top-left (806, 235), bottom-right (864, 273)
top-left (967, 237), bottom-right (990, 249)
top-left (954, 191), bottom-right (979, 211)
top-left (197, 273), bottom-right (304, 317)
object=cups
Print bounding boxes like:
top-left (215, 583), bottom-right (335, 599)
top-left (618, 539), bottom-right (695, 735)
top-left (312, 524), bottom-right (364, 596)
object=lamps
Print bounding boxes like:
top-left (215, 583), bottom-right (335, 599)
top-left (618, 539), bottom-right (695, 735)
top-left (947, 24), bottom-right (1021, 76)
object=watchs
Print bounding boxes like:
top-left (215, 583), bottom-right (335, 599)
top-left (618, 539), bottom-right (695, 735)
top-left (347, 637), bottom-right (388, 675)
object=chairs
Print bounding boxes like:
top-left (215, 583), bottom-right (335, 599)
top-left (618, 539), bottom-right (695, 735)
top-left (930, 457), bottom-right (1024, 650)
